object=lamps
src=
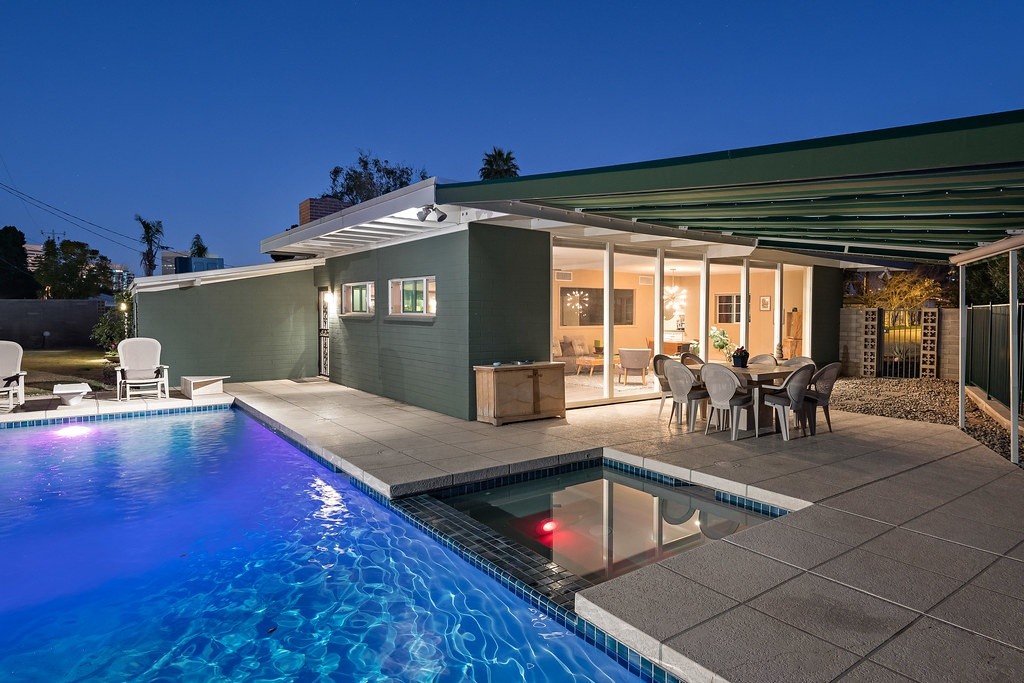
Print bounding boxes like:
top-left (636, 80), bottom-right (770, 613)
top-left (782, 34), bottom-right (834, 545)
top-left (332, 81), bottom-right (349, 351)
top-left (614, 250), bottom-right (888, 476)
top-left (416, 205), bottom-right (433, 222)
top-left (663, 269), bottom-right (687, 312)
top-left (567, 290), bottom-right (589, 318)
top-left (432, 206), bottom-right (447, 223)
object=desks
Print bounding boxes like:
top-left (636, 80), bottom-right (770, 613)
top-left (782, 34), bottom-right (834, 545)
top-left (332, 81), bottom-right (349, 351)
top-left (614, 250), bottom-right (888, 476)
top-left (649, 340), bottom-right (692, 356)
top-left (52, 383), bottom-right (92, 405)
top-left (685, 361), bottom-right (800, 431)
top-left (180, 376), bottom-right (231, 400)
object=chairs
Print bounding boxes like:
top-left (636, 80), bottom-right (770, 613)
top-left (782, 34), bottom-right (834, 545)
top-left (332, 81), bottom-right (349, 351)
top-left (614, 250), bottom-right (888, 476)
top-left (0, 340), bottom-right (28, 410)
top-left (646, 337), bottom-right (654, 347)
top-left (617, 348), bottom-right (652, 385)
top-left (652, 353), bottom-right (842, 442)
top-left (113, 336), bottom-right (170, 401)
top-left (660, 497), bottom-right (696, 525)
top-left (698, 509), bottom-right (740, 540)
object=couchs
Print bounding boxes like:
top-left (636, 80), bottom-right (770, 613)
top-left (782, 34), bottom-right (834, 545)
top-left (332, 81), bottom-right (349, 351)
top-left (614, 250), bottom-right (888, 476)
top-left (553, 335), bottom-right (594, 375)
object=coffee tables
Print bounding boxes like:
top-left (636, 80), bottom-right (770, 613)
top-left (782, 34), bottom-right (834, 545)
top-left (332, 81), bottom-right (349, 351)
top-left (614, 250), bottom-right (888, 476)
top-left (576, 354), bottom-right (621, 377)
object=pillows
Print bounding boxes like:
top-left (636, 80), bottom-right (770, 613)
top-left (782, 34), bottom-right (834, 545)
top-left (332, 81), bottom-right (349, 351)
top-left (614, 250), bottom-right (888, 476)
top-left (563, 336), bottom-right (575, 356)
top-left (571, 336), bottom-right (590, 355)
top-left (553, 336), bottom-right (563, 357)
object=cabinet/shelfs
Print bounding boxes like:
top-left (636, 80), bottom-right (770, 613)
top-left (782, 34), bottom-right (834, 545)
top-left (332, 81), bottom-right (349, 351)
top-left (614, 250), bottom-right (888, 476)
top-left (473, 361), bottom-right (566, 427)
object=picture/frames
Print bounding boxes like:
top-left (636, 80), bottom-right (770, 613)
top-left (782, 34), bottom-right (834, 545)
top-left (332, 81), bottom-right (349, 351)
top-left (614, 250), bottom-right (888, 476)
top-left (759, 296), bottom-right (771, 311)
